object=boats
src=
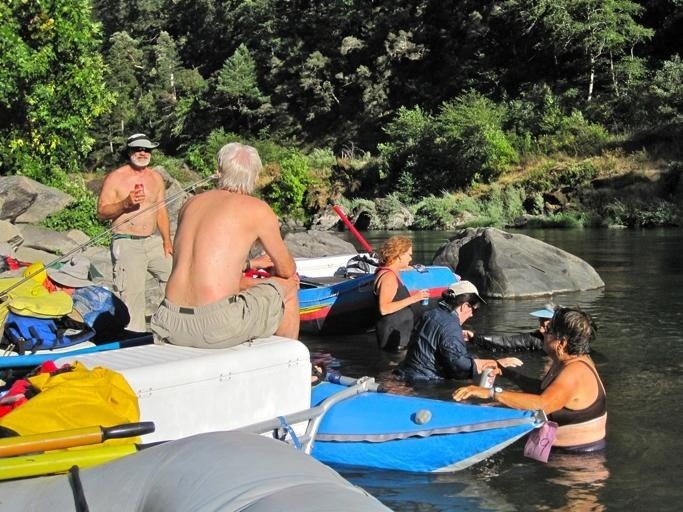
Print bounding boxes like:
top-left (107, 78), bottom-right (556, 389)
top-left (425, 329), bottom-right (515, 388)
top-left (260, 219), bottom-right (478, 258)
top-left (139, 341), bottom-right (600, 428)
top-left (290, 252), bottom-right (465, 335)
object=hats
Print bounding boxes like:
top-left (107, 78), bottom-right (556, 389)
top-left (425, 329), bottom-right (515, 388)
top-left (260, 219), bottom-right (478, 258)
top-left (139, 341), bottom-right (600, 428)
top-left (448, 280), bottom-right (487, 305)
top-left (128, 134), bottom-right (160, 149)
top-left (529, 308), bottom-right (554, 318)
top-left (47, 256), bottom-right (104, 288)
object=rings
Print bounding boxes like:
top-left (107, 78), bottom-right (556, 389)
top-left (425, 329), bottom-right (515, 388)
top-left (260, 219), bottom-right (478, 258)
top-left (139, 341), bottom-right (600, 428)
top-left (135, 198), bottom-right (138, 201)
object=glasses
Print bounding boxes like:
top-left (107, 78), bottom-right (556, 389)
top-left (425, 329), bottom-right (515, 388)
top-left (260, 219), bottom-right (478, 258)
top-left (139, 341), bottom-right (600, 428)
top-left (470, 305), bottom-right (479, 317)
top-left (134, 147), bottom-right (151, 153)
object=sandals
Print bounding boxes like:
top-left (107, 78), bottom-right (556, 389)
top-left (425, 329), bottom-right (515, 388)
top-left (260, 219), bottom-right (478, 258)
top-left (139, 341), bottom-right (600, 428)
top-left (312, 364), bottom-right (326, 386)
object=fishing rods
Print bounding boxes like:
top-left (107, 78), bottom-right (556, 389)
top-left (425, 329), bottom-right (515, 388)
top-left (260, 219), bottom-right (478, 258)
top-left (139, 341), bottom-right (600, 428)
top-left (0, 174), bottom-right (220, 295)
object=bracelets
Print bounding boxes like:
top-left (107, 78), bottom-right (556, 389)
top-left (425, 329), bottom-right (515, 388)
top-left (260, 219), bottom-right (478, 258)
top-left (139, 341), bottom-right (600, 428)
top-left (489, 386), bottom-right (503, 402)
top-left (244, 260), bottom-right (250, 272)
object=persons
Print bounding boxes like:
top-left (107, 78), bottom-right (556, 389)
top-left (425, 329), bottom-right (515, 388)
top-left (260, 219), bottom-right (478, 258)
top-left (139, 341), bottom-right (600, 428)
top-left (460, 302), bottom-right (607, 367)
top-left (149, 140), bottom-right (326, 387)
top-left (371, 233), bottom-right (431, 353)
top-left (95, 129), bottom-right (177, 334)
top-left (398, 279), bottom-right (523, 385)
top-left (448, 305), bottom-right (607, 455)
top-left (482, 441), bottom-right (611, 512)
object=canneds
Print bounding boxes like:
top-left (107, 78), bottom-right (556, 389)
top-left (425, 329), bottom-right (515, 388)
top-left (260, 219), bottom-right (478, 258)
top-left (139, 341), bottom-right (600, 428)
top-left (421, 288), bottom-right (430, 306)
top-left (135, 183), bottom-right (144, 195)
top-left (479, 367), bottom-right (497, 390)
